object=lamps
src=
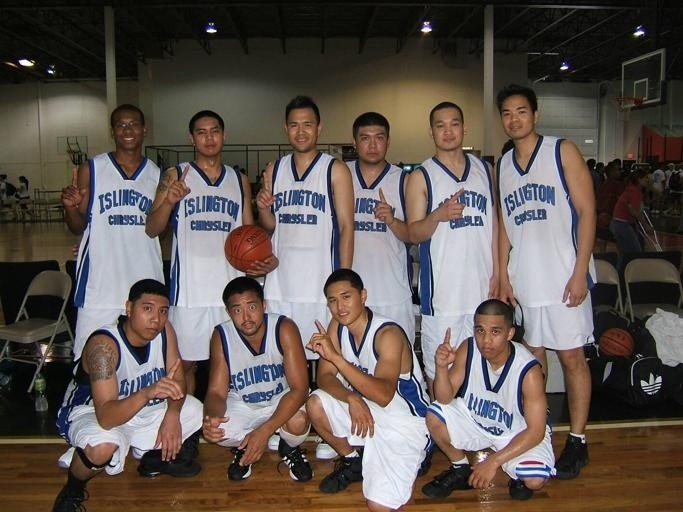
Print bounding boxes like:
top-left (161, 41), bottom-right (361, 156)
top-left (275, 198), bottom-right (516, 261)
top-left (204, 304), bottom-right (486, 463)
top-left (631, 24), bottom-right (647, 37)
top-left (17, 55), bottom-right (36, 68)
top-left (45, 64), bottom-right (57, 80)
top-left (560, 60), bottom-right (572, 73)
top-left (419, 20), bottom-right (433, 32)
top-left (204, 16), bottom-right (218, 33)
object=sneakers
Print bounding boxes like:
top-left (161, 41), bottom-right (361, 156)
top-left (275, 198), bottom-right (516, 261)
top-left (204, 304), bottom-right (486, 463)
top-left (268, 427), bottom-right (362, 493)
top-left (52, 483), bottom-right (89, 512)
top-left (227, 447), bottom-right (252, 481)
top-left (508, 478), bottom-right (533, 500)
top-left (417, 450), bottom-right (473, 498)
top-left (132, 430), bottom-right (201, 478)
top-left (58, 447), bottom-right (75, 469)
top-left (550, 434), bottom-right (589, 480)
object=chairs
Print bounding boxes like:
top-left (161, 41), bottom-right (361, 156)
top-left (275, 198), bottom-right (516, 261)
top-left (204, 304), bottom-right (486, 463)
top-left (0, 194), bottom-right (67, 222)
top-left (0, 269), bottom-right (73, 391)
top-left (594, 259), bottom-right (626, 316)
top-left (624, 258), bottom-right (683, 324)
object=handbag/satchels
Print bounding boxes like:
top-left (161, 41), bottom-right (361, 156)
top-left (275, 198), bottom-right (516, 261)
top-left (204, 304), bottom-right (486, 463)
top-left (585, 309), bottom-right (683, 421)
top-left (6, 182), bottom-right (16, 196)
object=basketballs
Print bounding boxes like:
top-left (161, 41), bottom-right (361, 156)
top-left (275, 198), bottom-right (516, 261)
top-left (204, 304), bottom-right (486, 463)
top-left (599, 327), bottom-right (635, 356)
top-left (225, 225), bottom-right (273, 271)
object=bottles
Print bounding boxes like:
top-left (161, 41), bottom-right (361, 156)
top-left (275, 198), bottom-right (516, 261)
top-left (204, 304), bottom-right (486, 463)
top-left (34, 373), bottom-right (48, 412)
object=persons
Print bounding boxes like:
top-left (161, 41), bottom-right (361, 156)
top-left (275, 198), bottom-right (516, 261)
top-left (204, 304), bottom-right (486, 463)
top-left (306, 268), bottom-right (435, 512)
top-left (422, 298), bottom-right (556, 501)
top-left (0, 174), bottom-right (34, 220)
top-left (145, 110), bottom-right (279, 395)
top-left (202, 276), bottom-right (314, 482)
top-left (494, 85), bottom-right (598, 480)
top-left (58, 103), bottom-right (166, 469)
top-left (405, 102), bottom-right (500, 400)
top-left (52, 279), bottom-right (204, 512)
top-left (345, 112), bottom-right (415, 350)
top-left (256, 96), bottom-right (354, 460)
top-left (586, 158), bottom-right (683, 312)
